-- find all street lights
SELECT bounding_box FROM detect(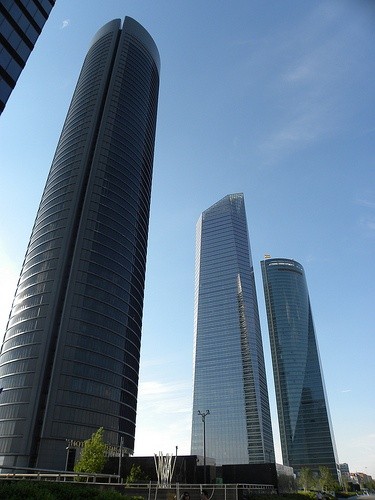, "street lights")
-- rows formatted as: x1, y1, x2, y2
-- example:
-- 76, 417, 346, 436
197, 408, 210, 484
265, 449, 272, 463
62, 445, 70, 481
